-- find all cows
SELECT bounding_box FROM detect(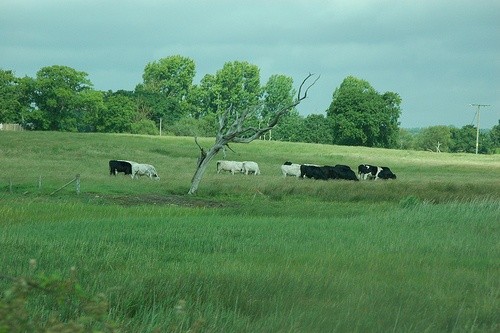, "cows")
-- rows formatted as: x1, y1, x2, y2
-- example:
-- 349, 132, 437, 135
109, 160, 161, 183
280, 161, 397, 181
216, 160, 261, 175
